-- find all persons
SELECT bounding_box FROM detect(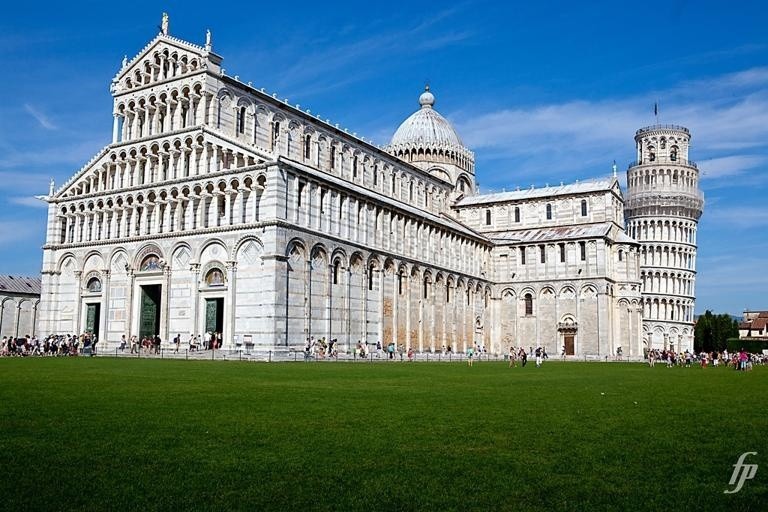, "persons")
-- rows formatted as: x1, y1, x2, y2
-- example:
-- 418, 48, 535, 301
468, 341, 486, 368
616, 347, 622, 361
356, 340, 413, 362
561, 346, 566, 361
2, 331, 97, 357
441, 345, 451, 355
509, 345, 548, 368
647, 349, 768, 372
117, 331, 222, 354
304, 336, 338, 359
